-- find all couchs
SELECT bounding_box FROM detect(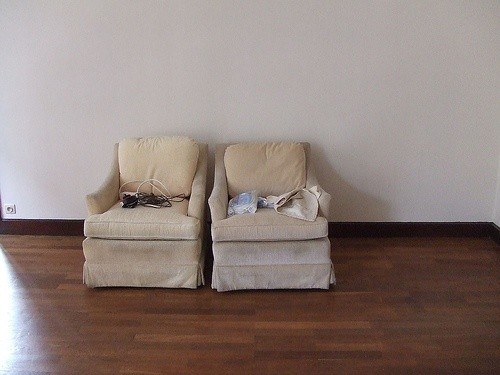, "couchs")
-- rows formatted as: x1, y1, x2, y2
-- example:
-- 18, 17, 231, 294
208, 142, 337, 292
82, 136, 208, 289
